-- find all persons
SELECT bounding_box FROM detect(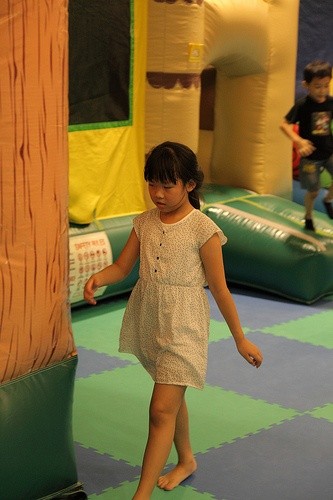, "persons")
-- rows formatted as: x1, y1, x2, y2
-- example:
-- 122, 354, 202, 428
83, 141, 265, 500
278, 58, 333, 233
